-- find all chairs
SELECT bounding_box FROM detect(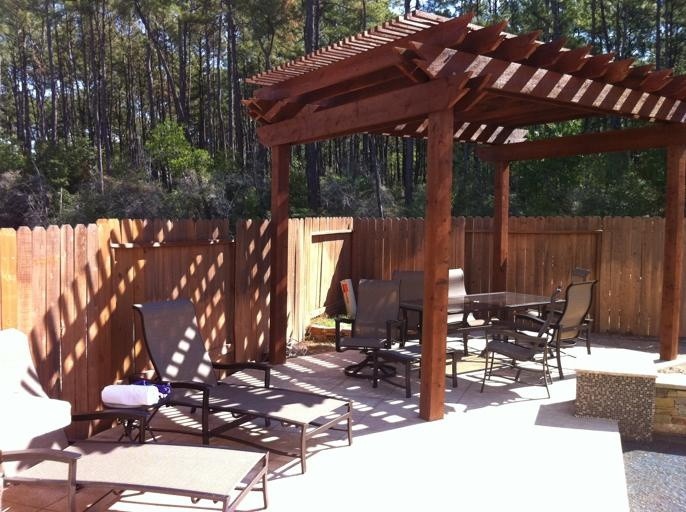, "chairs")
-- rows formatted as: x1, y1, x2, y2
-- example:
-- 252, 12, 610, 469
333, 267, 599, 400
